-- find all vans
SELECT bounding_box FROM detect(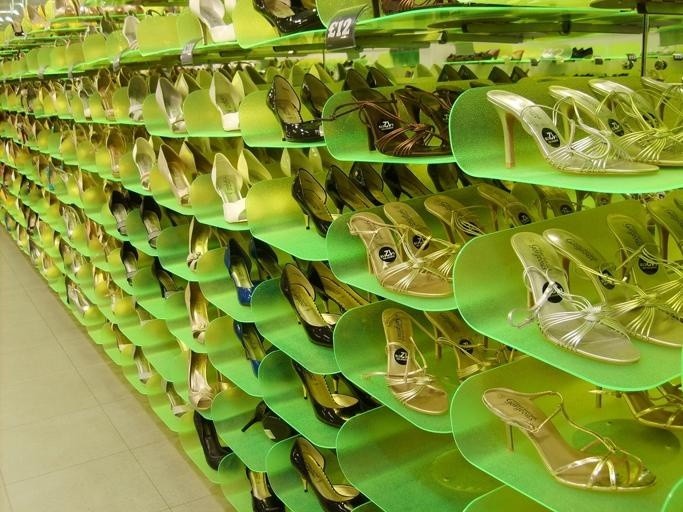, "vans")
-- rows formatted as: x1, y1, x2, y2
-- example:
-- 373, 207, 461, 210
439, 85, 464, 104
335, 65, 346, 82
157, 78, 188, 132
36, 154, 47, 175
223, 240, 257, 303
507, 233, 640, 364
281, 147, 313, 176
65, 209, 77, 237
238, 149, 271, 188
292, 362, 360, 428
75, 290, 90, 315
302, 75, 334, 118
397, 87, 452, 141
158, 145, 190, 207
429, 164, 459, 192
47, 167, 56, 190
306, 262, 372, 312
47, 162, 57, 190
133, 137, 155, 191
384, 201, 459, 281
413, 64, 432, 79
247, 473, 282, 512
97, 226, 116, 262
576, 192, 613, 209
26, 6, 47, 30
92, 265, 195, 298
65, 82, 78, 112
128, 77, 148, 121
425, 196, 489, 244
438, 86, 463, 106
194, 412, 233, 472
645, 203, 683, 249
483, 336, 520, 364
311, 146, 338, 171
134, 347, 153, 382
63, 207, 78, 241
139, 196, 161, 246
177, 74, 202, 95
323, 165, 374, 213
46, 1, 68, 29
250, 238, 284, 282
99, 18, 115, 37
187, 350, 214, 412
168, 211, 186, 227
6, 142, 17, 163
188, 218, 212, 273
26, 87, 36, 111
218, 373, 234, 392
331, 371, 380, 410
117, 66, 133, 87
267, 75, 326, 144
384, 163, 432, 201
209, 73, 244, 130
282, 264, 342, 347
254, 1, 319, 32
368, 68, 391, 87
17, 226, 26, 245
10, 21, 23, 36
28, 211, 37, 236
39, 88, 49, 103
30, 241, 42, 266
212, 154, 247, 224
112, 325, 129, 351
351, 163, 391, 207
107, 276, 122, 312
372, 0, 463, 17
79, 77, 95, 118
179, 141, 213, 176
265, 67, 283, 83
3, 166, 11, 187
484, 389, 656, 492
546, 228, 683, 347
291, 437, 367, 512
42, 253, 53, 275
319, 90, 450, 157
290, 170, 341, 237
348, 212, 452, 297
150, 258, 182, 299
292, 0, 316, 12
477, 183, 535, 231
107, 128, 126, 178
288, 65, 306, 87
245, 67, 266, 85
231, 71, 258, 98
608, 216, 683, 317
591, 77, 682, 146
78, 175, 92, 198
547, 84, 683, 168
95, 66, 117, 118
308, 65, 334, 84
109, 192, 128, 236
468, 81, 492, 90
122, 15, 142, 50
5, 212, 13, 230
366, 308, 450, 413
71, 252, 83, 274
162, 380, 189, 419
457, 65, 478, 79
33, 121, 43, 141
133, 300, 152, 327
511, 67, 529, 81
437, 65, 461, 82
488, 68, 513, 85
190, 1, 236, 45
593, 386, 682, 430
422, 310, 500, 382
186, 281, 209, 344
234, 319, 265, 378
487, 91, 658, 174
224, 0, 237, 16
120, 242, 138, 288
341, 69, 372, 89
643, 78, 683, 120
242, 402, 293, 442
125, 190, 142, 211
658, 384, 682, 403
534, 185, 577, 218
214, 226, 243, 244
74, 124, 87, 151
